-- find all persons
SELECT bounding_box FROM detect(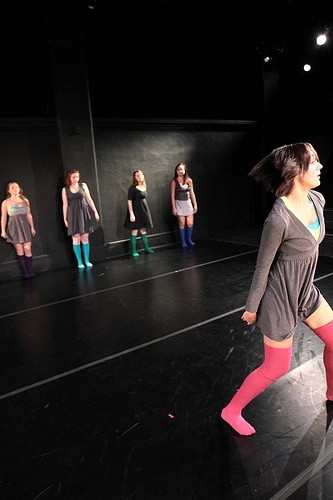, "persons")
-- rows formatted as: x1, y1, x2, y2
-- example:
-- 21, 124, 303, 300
124, 170, 155, 256
1, 182, 35, 279
222, 144, 333, 434
62, 169, 100, 268
171, 163, 198, 246
